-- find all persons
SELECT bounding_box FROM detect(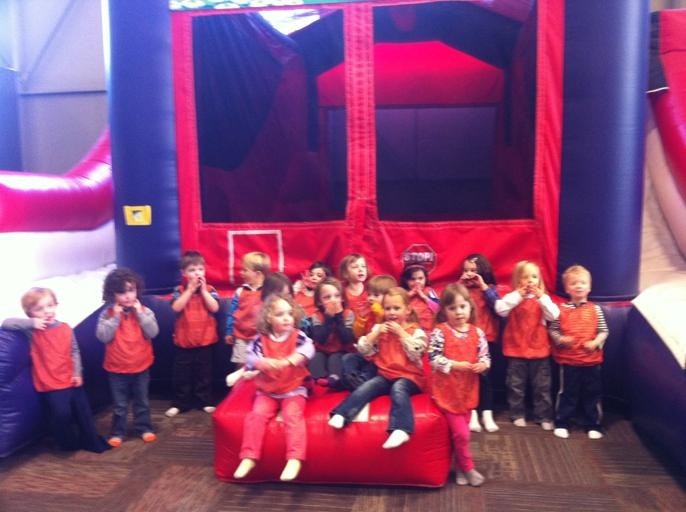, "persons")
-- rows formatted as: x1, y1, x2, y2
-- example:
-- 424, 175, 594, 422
95, 268, 160, 448
327, 287, 431, 449
340, 274, 397, 390
426, 283, 491, 487
164, 251, 221, 418
233, 294, 315, 481
307, 277, 356, 390
259, 272, 310, 333
459, 253, 502, 433
401, 261, 440, 332
336, 252, 370, 336
292, 261, 331, 323
548, 265, 609, 439
225, 252, 270, 371
494, 259, 560, 430
2, 287, 112, 453
